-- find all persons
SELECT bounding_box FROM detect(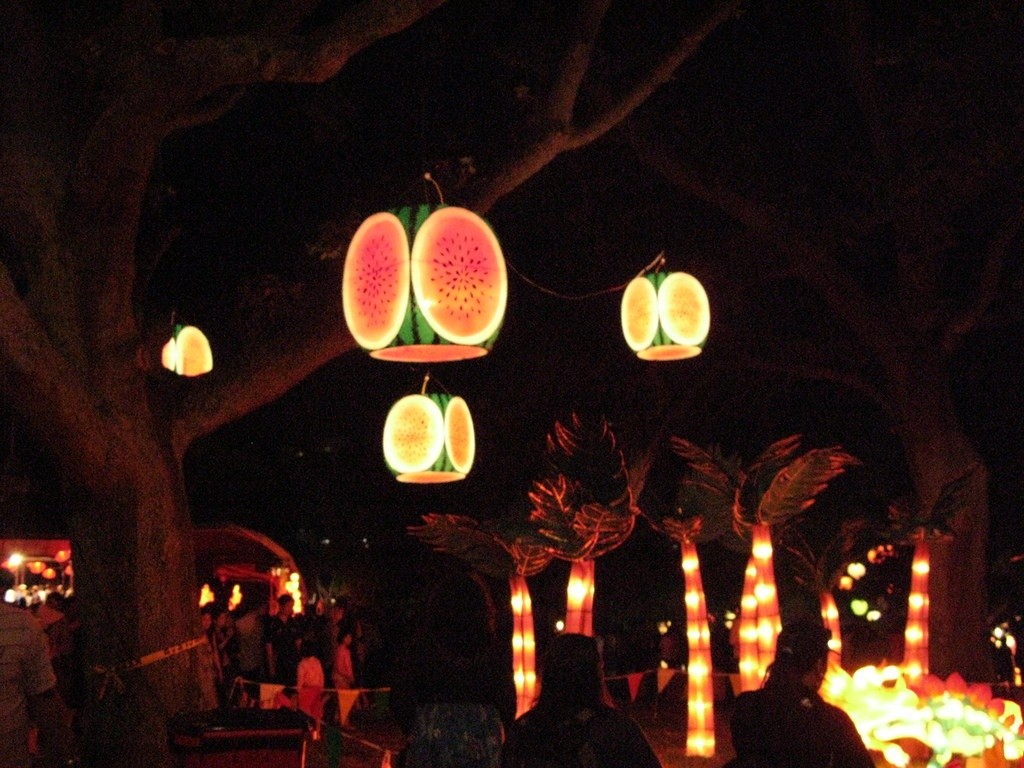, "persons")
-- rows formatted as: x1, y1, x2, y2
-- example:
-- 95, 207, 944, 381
498, 633, 666, 768
0, 568, 366, 767
728, 623, 873, 767
